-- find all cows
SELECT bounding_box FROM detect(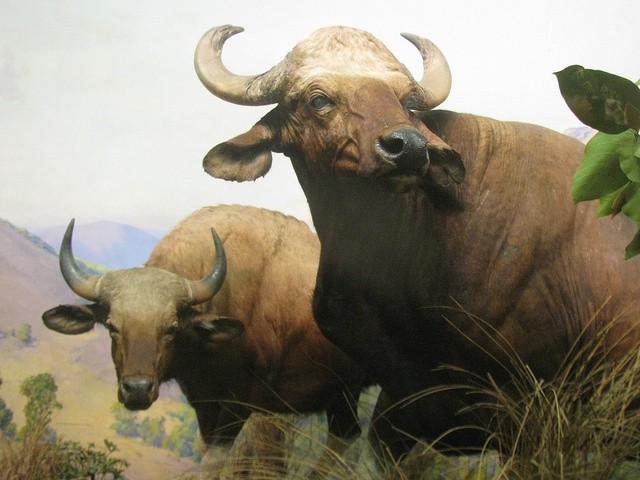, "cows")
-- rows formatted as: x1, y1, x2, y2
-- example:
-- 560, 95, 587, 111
194, 25, 640, 471
41, 204, 377, 480
